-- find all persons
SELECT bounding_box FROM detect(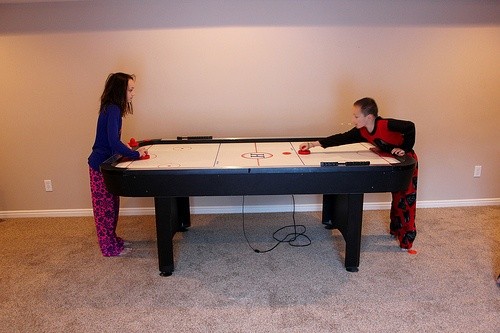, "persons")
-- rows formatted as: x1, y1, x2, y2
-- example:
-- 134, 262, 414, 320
300, 96, 421, 252
86, 71, 150, 258
